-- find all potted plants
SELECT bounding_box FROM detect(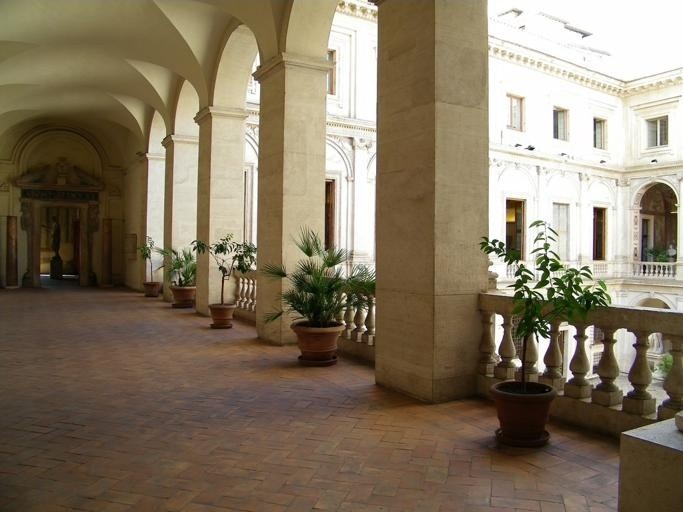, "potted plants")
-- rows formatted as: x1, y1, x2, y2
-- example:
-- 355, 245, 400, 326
475, 220, 612, 447
132, 230, 377, 368
